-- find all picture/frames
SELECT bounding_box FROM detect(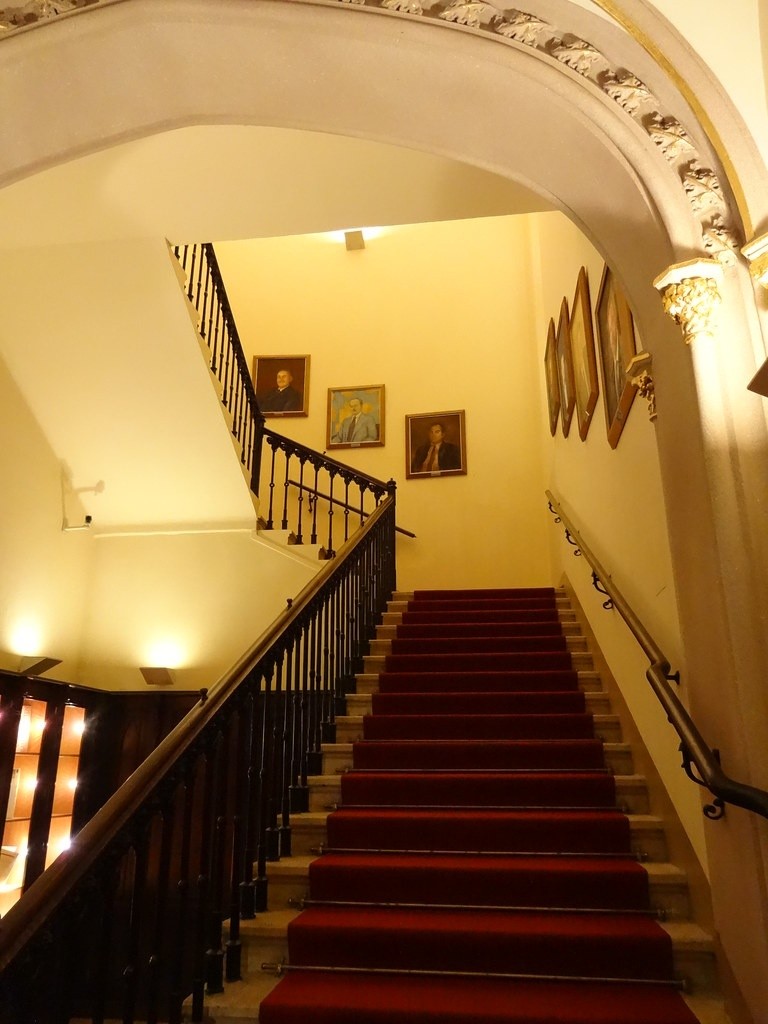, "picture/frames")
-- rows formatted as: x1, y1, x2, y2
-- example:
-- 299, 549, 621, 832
553, 296, 576, 438
405, 409, 467, 479
568, 265, 599, 442
250, 354, 310, 417
543, 318, 561, 436
594, 261, 638, 449
326, 384, 386, 449
